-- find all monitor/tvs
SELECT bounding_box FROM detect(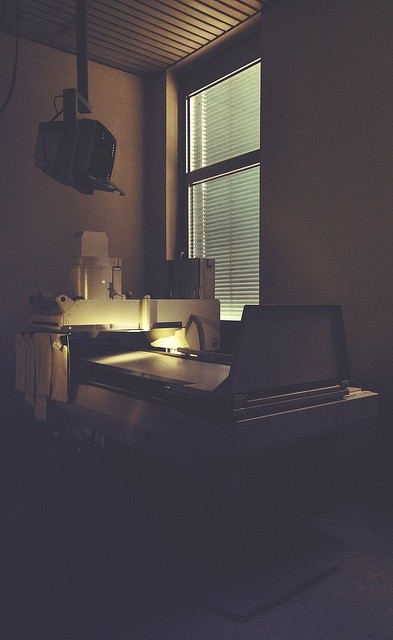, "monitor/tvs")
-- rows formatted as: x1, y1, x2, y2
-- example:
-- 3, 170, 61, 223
33, 118, 118, 183
